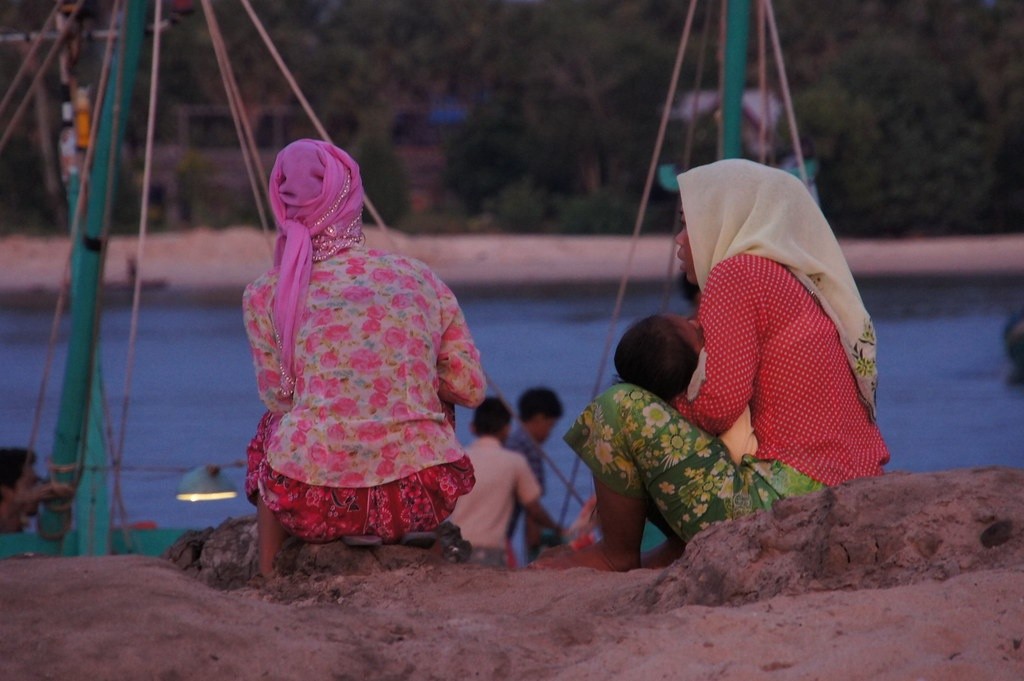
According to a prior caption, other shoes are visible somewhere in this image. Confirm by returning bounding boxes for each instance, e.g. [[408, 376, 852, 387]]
[[403, 530, 437, 548], [343, 534, 383, 547]]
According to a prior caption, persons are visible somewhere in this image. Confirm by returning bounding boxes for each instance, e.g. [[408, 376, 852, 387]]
[[528, 157, 889, 573], [1003, 310, 1024, 384], [507, 386, 563, 496], [0, 448, 39, 534], [445, 397, 569, 569], [613, 314, 757, 465], [244, 139, 489, 546]]
